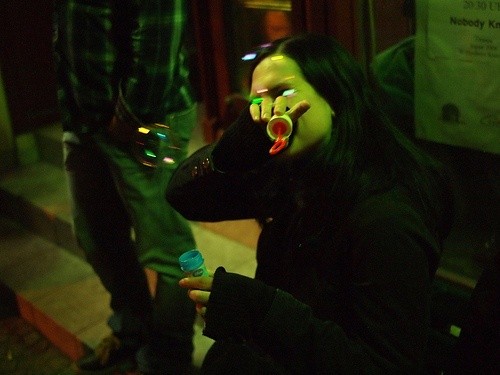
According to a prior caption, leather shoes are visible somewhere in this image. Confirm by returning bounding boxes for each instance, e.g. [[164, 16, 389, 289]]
[[77, 334, 137, 370]]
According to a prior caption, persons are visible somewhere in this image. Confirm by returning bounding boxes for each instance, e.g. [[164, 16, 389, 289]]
[[164, 29, 455, 375], [52, 0, 198, 375]]
